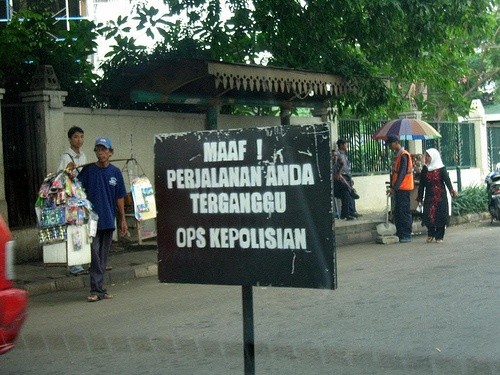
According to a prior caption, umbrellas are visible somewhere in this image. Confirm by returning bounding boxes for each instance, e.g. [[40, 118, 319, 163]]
[[372, 115, 442, 140]]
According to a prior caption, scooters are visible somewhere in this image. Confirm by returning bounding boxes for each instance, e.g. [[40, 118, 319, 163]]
[[484, 163, 500, 224]]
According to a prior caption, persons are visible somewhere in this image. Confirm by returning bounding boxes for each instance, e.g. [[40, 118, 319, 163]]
[[57, 125, 89, 276], [386, 135, 414, 243], [331, 138, 363, 221], [66, 137, 127, 302], [417, 148, 456, 243]]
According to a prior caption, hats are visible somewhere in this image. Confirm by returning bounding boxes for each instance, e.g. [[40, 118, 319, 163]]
[[96, 137, 112, 148]]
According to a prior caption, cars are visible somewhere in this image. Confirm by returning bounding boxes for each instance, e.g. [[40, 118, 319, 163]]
[[0, 213, 30, 357]]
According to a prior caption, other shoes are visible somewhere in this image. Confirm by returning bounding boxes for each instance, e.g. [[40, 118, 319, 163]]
[[88, 295, 97, 301], [427, 237, 433, 241], [437, 239, 440, 242], [104, 292, 113, 298]]
